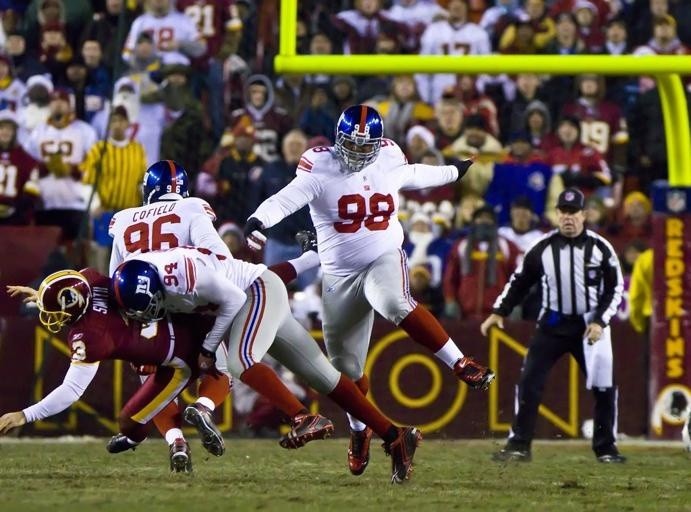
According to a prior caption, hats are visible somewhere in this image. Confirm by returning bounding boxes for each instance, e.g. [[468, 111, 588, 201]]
[[553, 187, 585, 210]]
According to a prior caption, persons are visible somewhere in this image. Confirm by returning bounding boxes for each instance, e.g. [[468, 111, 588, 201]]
[[479, 186, 628, 464], [241, 103, 495, 476], [110, 246, 422, 484], [2, 225, 321, 457], [0, 0, 691, 322], [107, 158, 234, 473]]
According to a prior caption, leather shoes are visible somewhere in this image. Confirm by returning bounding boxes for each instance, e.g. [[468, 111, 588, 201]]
[[491, 448, 531, 463], [597, 454, 626, 466]]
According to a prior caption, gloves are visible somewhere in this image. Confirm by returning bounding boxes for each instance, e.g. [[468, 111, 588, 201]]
[[243, 215, 267, 252], [452, 153, 477, 183]]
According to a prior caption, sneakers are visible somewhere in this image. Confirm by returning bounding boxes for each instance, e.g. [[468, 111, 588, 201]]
[[346, 420, 373, 475], [380, 425, 422, 486], [169, 437, 192, 474], [105, 433, 143, 453], [294, 225, 318, 252], [452, 354, 496, 392], [185, 400, 228, 459], [277, 413, 335, 450]]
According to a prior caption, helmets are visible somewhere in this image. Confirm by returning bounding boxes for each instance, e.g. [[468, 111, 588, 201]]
[[36, 269, 94, 334], [111, 259, 168, 323], [140, 159, 191, 202], [332, 104, 384, 173]]
